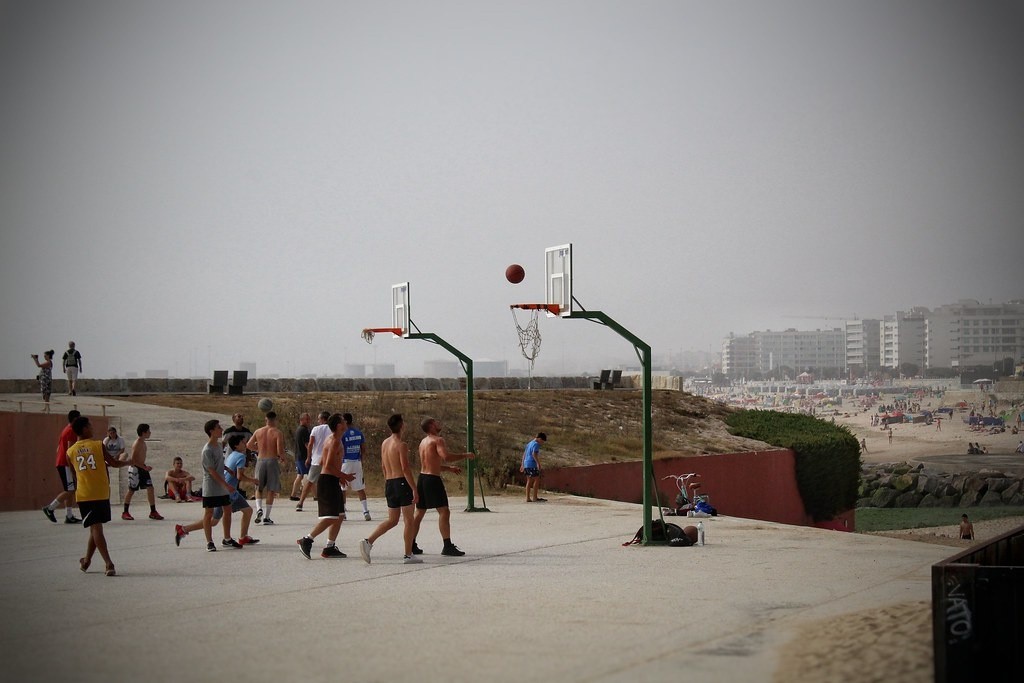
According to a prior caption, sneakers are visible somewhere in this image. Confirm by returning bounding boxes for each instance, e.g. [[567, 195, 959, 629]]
[[360, 538, 373, 564], [122, 511, 134, 520], [296, 535, 314, 559], [105, 565, 115, 576], [403, 554, 423, 564], [63, 515, 83, 524], [175, 508, 274, 552], [42, 506, 57, 523], [321, 543, 346, 558], [149, 511, 164, 520], [80, 558, 88, 572], [412, 547, 423, 554], [441, 543, 465, 556]]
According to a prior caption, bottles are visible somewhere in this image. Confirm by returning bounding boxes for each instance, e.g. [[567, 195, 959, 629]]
[[698, 521, 704, 545], [694, 496, 698, 504]]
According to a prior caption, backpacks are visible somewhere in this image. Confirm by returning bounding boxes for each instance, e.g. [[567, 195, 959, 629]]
[[65, 350, 77, 365]]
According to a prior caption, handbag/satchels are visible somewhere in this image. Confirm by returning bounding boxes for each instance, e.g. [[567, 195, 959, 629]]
[[664, 522, 692, 547], [622, 519, 667, 546]]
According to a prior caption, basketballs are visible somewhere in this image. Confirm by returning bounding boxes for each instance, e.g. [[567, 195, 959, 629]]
[[505, 264, 525, 284], [258, 397, 273, 413]]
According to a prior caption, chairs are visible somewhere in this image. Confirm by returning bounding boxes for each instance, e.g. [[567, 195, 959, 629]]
[[591, 370, 611, 390], [207, 370, 228, 394], [602, 370, 622, 390], [227, 370, 249, 394]]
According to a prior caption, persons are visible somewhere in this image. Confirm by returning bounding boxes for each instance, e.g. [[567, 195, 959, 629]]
[[102, 427, 128, 467], [859, 384, 1024, 456], [246, 412, 285, 525], [519, 432, 548, 503], [164, 456, 195, 503], [176, 434, 260, 547], [66, 416, 138, 576], [411, 417, 475, 557], [62, 341, 83, 396], [359, 414, 423, 564], [289, 412, 319, 501], [295, 410, 334, 511], [222, 412, 252, 460], [343, 412, 371, 521], [41, 410, 82, 525], [121, 424, 164, 521], [30, 349, 55, 413], [202, 420, 243, 552], [959, 514, 974, 540], [297, 412, 357, 560]]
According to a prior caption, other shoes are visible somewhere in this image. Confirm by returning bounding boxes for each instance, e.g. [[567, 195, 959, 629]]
[[176, 495, 181, 503], [364, 512, 371, 521], [69, 393, 71, 395], [289, 496, 300, 501], [314, 497, 318, 500], [296, 504, 302, 510], [72, 388, 75, 396], [185, 495, 193, 501]]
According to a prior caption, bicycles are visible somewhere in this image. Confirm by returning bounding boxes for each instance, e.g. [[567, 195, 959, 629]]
[[662, 472, 710, 505]]
